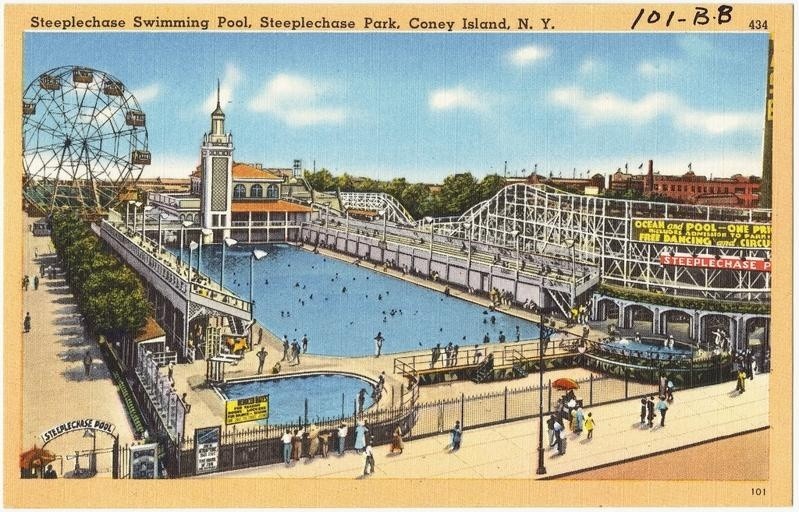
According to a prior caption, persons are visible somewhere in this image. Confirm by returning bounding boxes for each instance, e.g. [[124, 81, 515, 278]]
[[23, 218, 746, 479]]
